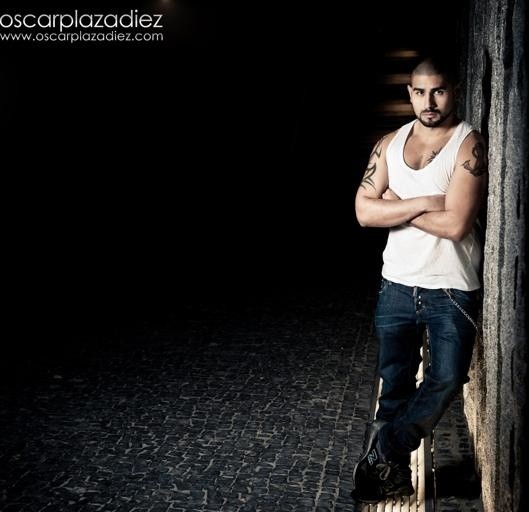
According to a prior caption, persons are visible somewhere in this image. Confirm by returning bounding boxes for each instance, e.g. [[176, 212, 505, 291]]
[[352, 58, 487, 504]]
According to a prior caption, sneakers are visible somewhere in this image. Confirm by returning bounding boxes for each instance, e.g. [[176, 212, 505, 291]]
[[353, 419, 392, 487], [350, 465, 415, 503]]
